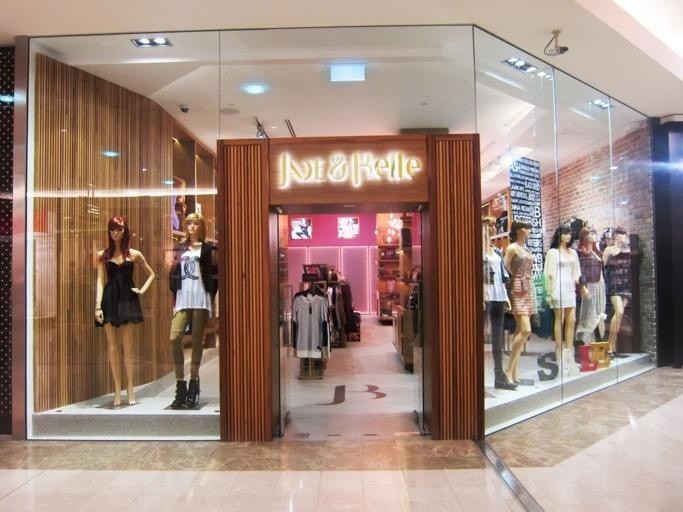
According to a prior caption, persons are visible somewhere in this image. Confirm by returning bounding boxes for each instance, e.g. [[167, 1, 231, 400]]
[[503, 220, 538, 388], [95, 217, 155, 406], [482, 217, 519, 390], [576, 226, 606, 345], [544, 225, 591, 377], [602, 228, 633, 357], [169, 214, 218, 408]]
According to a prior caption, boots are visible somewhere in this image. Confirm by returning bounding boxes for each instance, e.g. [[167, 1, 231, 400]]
[[494, 367, 518, 390], [180, 378, 200, 409], [171, 380, 188, 408]]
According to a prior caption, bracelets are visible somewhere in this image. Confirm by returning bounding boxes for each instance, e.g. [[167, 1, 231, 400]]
[[95, 308, 102, 313]]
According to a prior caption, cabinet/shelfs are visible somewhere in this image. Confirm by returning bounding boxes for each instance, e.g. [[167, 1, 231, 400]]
[[401, 265, 423, 346], [169, 118, 220, 345], [377, 244, 399, 320], [481, 186, 512, 241]]
